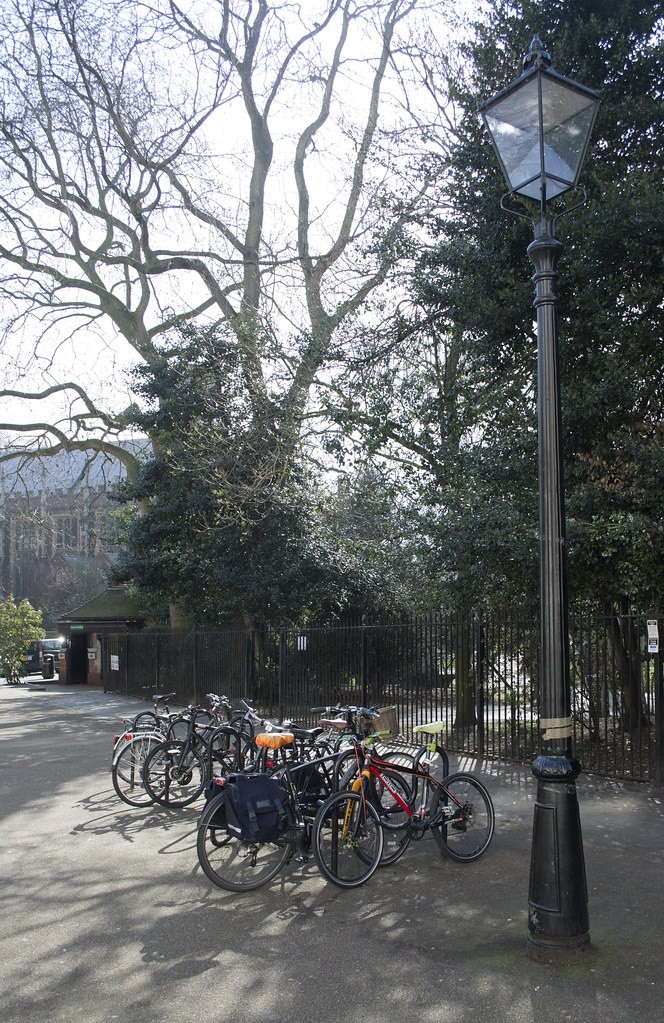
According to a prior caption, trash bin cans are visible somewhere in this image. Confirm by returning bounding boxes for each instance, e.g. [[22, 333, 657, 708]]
[[42, 653, 55, 679]]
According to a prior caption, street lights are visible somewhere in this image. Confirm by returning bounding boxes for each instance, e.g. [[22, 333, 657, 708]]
[[473, 33, 609, 964]]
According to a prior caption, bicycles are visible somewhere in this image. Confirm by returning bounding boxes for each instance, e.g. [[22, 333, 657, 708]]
[[107, 693, 499, 896]]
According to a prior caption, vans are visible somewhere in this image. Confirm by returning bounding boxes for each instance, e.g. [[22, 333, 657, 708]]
[[19, 639, 63, 672]]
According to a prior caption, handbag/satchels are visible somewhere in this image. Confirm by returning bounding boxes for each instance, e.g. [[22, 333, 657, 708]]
[[202, 779, 224, 825], [223, 776, 293, 843]]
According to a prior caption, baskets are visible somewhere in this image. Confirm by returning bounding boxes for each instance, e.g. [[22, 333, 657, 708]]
[[360, 706, 400, 740]]
